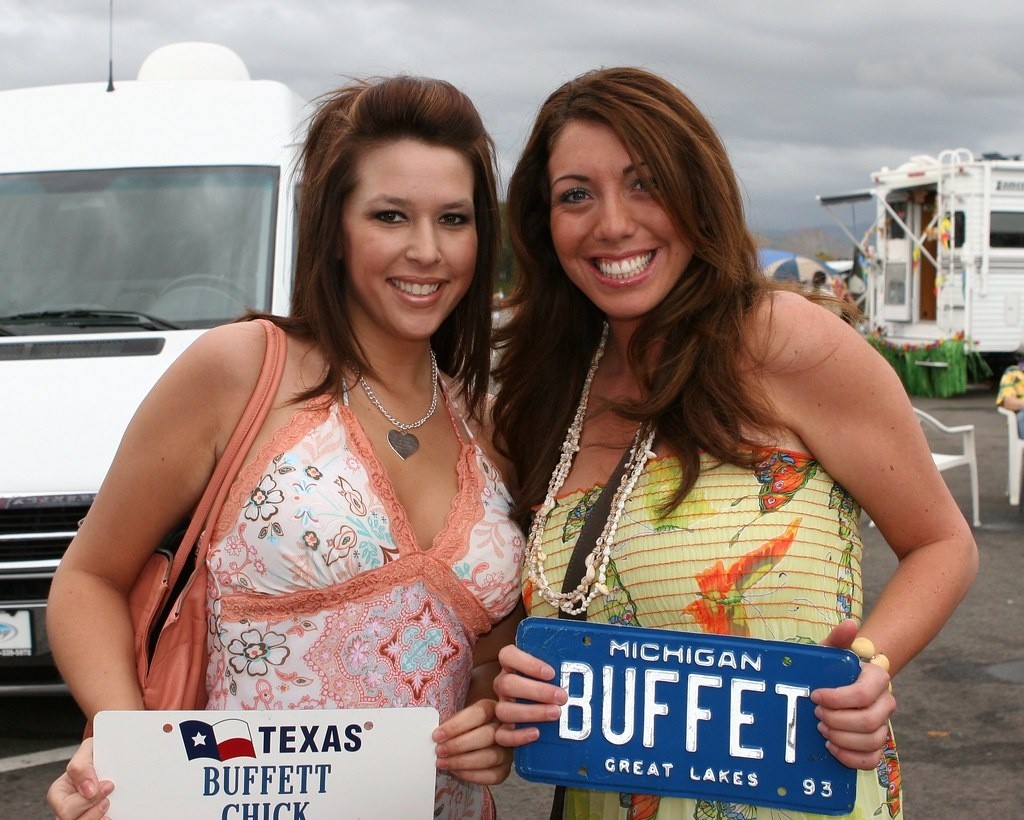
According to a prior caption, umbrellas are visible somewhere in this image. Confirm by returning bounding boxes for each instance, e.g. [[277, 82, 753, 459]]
[[750, 245, 843, 294]]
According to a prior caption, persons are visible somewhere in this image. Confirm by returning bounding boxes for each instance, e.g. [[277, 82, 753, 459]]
[[493, 65, 981, 820], [995, 342, 1024, 442], [44, 76, 530, 818], [810, 270, 841, 316]]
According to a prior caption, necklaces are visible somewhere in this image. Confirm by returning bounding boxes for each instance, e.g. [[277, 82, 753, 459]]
[[345, 344, 441, 460], [519, 317, 654, 617]]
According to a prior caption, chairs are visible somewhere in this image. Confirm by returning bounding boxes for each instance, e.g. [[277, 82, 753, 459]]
[[868, 408, 983, 527], [996, 405, 1024, 504]]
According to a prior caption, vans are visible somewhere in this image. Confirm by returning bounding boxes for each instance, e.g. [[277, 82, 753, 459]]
[[2, 40, 520, 695]]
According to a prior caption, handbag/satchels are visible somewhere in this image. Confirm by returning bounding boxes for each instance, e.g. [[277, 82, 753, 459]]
[[82, 318, 286, 746]]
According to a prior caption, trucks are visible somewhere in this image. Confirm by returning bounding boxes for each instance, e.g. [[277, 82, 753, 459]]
[[815, 149, 1024, 391]]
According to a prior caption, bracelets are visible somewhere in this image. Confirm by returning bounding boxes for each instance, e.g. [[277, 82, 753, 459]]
[[846, 635, 897, 703]]
